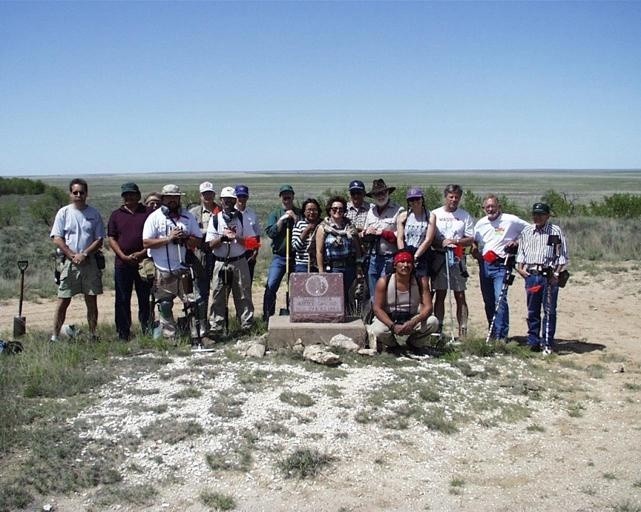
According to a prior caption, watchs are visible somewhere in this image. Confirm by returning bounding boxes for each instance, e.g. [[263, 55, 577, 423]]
[[83, 252, 88, 257]]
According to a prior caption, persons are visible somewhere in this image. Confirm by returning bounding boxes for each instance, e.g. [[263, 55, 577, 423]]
[[261, 178, 475, 356], [470, 196, 531, 341], [516, 203, 566, 355], [50, 178, 106, 343], [105, 182, 261, 346]]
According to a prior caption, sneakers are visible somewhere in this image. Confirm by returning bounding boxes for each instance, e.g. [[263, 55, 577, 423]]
[[539, 346, 552, 356], [192, 336, 214, 347]]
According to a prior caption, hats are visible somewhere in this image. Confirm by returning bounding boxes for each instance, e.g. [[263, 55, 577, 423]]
[[532, 202, 549, 214], [348, 180, 364, 191], [279, 185, 295, 196], [406, 187, 423, 199], [120, 182, 250, 204], [365, 178, 396, 198]]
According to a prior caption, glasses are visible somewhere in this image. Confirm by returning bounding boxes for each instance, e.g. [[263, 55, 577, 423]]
[[409, 197, 420, 202], [73, 191, 85, 195], [373, 190, 386, 196], [332, 207, 344, 212], [350, 189, 361, 195]]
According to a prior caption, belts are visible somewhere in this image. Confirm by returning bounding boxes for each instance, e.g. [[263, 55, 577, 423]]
[[528, 264, 548, 273], [218, 255, 244, 261]]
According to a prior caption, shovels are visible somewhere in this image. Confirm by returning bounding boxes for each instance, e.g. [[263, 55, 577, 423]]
[[13, 260, 29, 339], [279, 219, 290, 316], [177, 303, 190, 332]]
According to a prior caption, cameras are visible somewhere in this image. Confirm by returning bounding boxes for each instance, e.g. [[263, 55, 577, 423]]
[[392, 312, 410, 324], [173, 231, 189, 243], [406, 245, 418, 254]]
